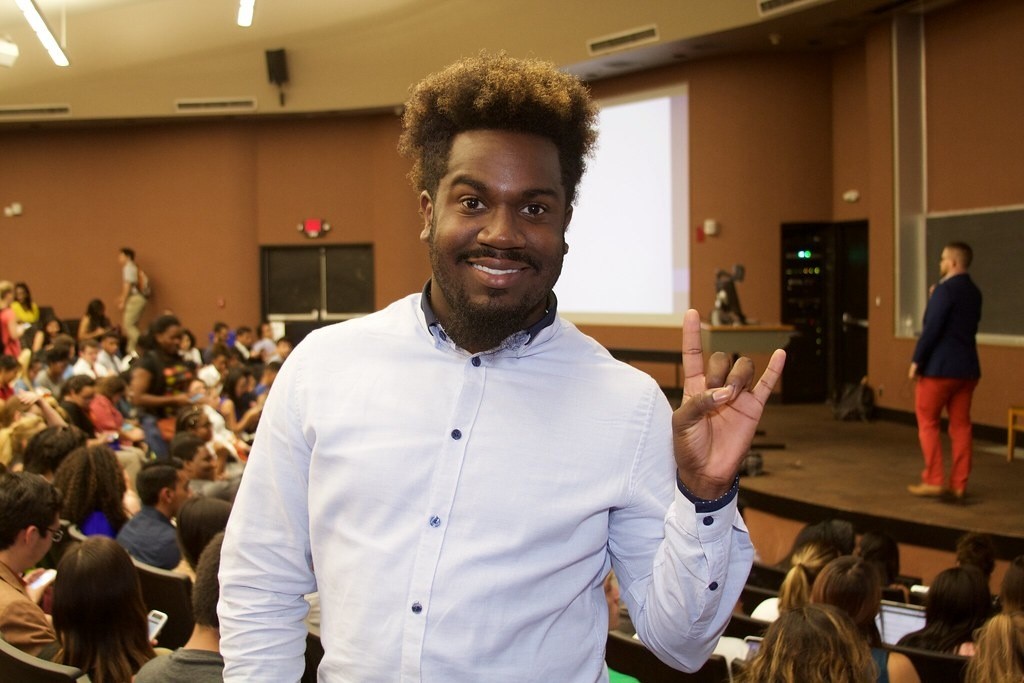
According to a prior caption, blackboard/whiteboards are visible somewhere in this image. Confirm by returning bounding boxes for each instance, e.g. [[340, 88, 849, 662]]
[[916, 204, 1023, 342]]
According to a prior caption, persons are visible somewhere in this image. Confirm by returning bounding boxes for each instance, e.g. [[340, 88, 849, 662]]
[[907, 241, 982, 498], [732, 520, 1024, 683], [1, 280, 292, 682], [604, 573, 641, 683], [44, 535, 177, 683], [117, 248, 148, 350], [117, 459, 193, 570], [217, 50, 786, 683]]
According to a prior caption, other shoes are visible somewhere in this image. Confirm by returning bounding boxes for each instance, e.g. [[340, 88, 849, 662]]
[[946, 485, 966, 501], [906, 481, 946, 497]]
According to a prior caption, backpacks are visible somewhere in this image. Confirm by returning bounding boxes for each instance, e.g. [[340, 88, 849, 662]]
[[132, 266, 152, 298]]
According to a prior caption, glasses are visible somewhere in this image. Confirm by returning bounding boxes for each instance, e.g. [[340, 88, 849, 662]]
[[40, 527, 65, 541]]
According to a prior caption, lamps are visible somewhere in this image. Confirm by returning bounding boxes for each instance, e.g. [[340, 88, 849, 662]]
[[14, 0, 70, 66], [295, 219, 330, 238]]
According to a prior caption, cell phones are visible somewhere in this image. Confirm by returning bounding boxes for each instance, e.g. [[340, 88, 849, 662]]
[[27, 568, 58, 590], [146, 609, 168, 641]]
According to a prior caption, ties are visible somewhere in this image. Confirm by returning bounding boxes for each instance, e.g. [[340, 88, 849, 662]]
[[90, 364, 98, 378], [110, 354, 122, 374]]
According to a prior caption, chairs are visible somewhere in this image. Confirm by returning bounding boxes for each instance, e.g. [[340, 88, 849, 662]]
[[1, 516, 973, 683], [1008, 407, 1024, 461]]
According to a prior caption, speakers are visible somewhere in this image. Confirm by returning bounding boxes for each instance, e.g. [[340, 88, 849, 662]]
[[267, 48, 287, 84]]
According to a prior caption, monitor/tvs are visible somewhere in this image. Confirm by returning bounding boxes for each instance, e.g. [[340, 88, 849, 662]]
[[716, 271, 745, 323]]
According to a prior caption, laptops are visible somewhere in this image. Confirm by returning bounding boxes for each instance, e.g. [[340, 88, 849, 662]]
[[874, 599, 928, 646]]
